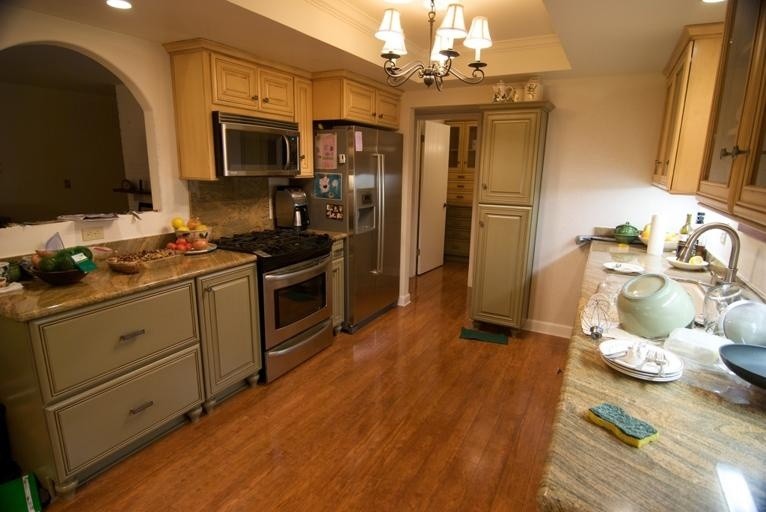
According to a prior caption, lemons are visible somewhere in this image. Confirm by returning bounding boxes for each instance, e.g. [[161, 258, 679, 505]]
[[172, 217, 207, 231]]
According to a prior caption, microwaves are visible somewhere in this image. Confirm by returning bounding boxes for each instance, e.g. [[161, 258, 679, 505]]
[[211, 110, 300, 178]]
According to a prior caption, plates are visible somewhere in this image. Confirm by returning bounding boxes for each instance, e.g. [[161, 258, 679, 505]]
[[665, 257, 708, 271], [638, 235, 677, 252], [183, 243, 217, 255], [598, 340, 684, 382], [603, 263, 644, 275]]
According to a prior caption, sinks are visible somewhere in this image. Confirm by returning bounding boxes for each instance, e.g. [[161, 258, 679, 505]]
[[664, 277, 707, 316]]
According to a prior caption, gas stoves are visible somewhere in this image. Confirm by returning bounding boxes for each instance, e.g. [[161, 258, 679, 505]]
[[207, 228, 334, 263]]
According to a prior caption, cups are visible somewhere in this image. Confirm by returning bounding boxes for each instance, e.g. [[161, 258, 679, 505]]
[[646, 215, 667, 255]]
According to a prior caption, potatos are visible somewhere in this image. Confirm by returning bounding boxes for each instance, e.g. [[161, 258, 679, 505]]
[[192, 240, 208, 249]]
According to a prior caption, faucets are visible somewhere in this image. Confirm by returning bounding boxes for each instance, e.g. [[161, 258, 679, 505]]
[[679, 222, 741, 281]]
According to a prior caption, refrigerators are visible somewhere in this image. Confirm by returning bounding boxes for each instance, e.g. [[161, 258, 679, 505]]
[[307, 124, 402, 334]]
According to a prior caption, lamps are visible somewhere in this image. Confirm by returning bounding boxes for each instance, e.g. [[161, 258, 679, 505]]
[[375, 1, 495, 94]]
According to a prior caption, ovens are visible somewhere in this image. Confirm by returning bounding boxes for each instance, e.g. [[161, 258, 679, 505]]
[[256, 256, 332, 384]]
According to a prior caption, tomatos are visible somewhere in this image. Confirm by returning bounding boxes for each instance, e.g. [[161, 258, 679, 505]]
[[167, 238, 192, 251]]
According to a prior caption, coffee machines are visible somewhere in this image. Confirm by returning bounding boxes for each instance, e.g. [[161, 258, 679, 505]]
[[274, 185, 309, 231]]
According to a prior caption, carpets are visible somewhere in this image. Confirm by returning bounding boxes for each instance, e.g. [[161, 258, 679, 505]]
[[459, 327, 509, 345]]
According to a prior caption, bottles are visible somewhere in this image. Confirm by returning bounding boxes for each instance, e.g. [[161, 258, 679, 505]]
[[692, 210, 706, 260], [676, 213, 693, 258]]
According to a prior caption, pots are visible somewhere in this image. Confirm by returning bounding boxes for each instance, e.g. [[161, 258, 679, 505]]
[[719, 344, 766, 390]]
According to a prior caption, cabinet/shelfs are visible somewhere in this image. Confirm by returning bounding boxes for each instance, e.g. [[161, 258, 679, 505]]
[[445, 206, 473, 257], [0, 279, 204, 501], [652, 21, 726, 198], [195, 261, 264, 417], [295, 65, 315, 180], [312, 69, 403, 131], [467, 203, 538, 333], [696, 1, 766, 233], [161, 36, 295, 122], [474, 101, 556, 207], [443, 119, 480, 208]]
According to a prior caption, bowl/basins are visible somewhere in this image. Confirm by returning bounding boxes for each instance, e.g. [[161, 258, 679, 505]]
[[30, 265, 88, 285], [617, 273, 695, 338], [703, 284, 752, 337], [613, 234, 637, 245], [0, 261, 29, 288], [174, 226, 212, 245]]
